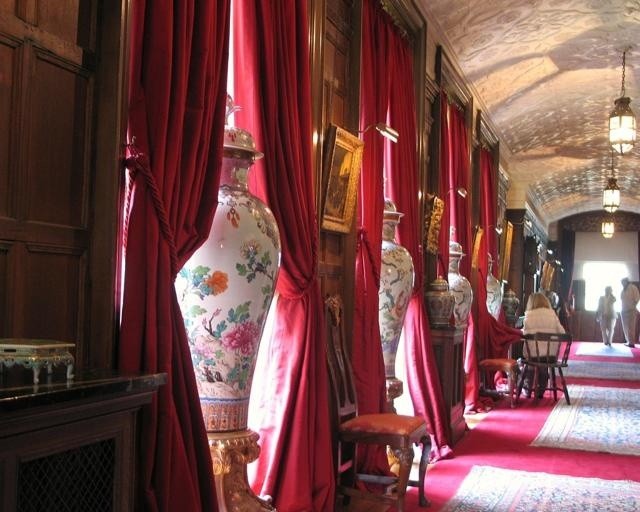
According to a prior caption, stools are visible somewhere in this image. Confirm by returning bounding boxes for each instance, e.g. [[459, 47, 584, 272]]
[[477, 358, 518, 409]]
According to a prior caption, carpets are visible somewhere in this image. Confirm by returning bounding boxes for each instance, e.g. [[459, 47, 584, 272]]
[[549, 359, 640, 381], [528, 383, 640, 456], [576, 340, 634, 358], [438, 464, 640, 511]]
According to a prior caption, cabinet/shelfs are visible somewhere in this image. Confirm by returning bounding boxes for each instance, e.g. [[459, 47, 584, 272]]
[[431, 327, 467, 446]]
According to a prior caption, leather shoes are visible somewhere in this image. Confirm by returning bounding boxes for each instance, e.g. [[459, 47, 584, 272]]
[[625, 343, 634, 347]]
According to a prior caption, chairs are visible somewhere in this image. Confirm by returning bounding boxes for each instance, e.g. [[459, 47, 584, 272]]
[[319, 292, 431, 511], [519, 332, 573, 408]]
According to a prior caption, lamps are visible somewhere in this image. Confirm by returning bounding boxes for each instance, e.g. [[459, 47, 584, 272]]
[[601, 215, 616, 240], [602, 147, 621, 215], [609, 51, 636, 157], [426, 187, 468, 198], [473, 224, 502, 236], [524, 217, 568, 273], [343, 120, 401, 144]]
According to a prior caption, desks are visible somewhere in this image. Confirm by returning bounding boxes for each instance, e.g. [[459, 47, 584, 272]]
[[0, 370, 169, 512]]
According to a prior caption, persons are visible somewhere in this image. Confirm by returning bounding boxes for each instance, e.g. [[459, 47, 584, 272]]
[[594, 286, 618, 348], [620, 277, 640, 349], [518, 291, 565, 397]]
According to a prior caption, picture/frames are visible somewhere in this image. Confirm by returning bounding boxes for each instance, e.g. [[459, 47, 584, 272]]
[[470, 224, 484, 271], [422, 192, 445, 257], [500, 220, 513, 286], [540, 261, 555, 291], [318, 122, 366, 235]]
